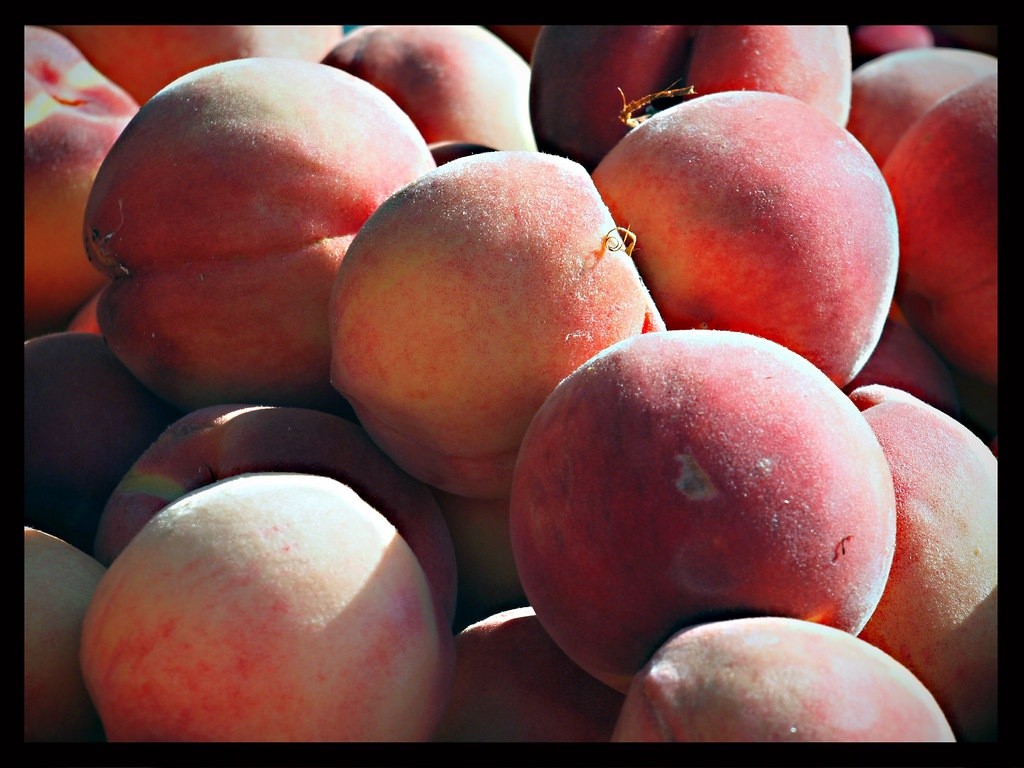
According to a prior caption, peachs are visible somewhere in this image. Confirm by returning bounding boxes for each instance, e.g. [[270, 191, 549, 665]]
[[25, 25, 998, 743]]
